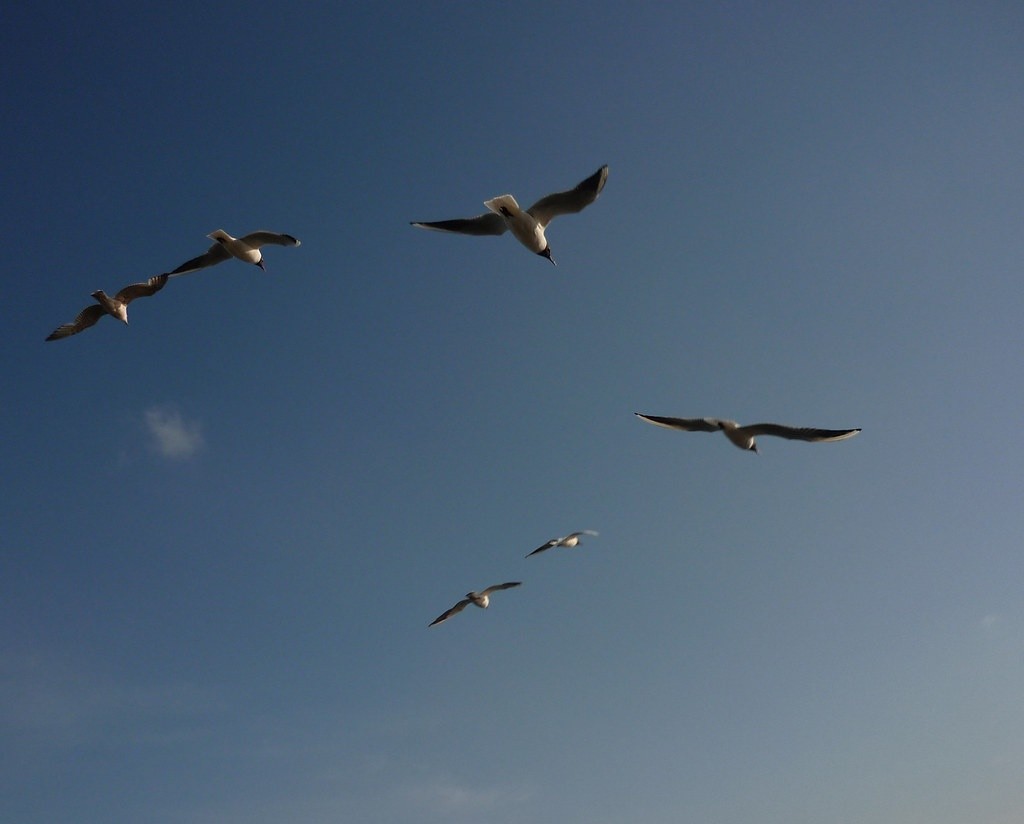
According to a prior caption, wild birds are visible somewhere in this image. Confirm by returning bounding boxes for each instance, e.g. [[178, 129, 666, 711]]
[[167, 228, 303, 273], [408, 163, 608, 267], [524, 530, 600, 559], [634, 412, 863, 456], [44, 273, 169, 342], [428, 581, 523, 627]]
